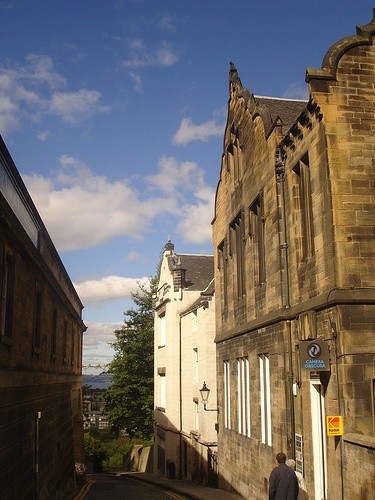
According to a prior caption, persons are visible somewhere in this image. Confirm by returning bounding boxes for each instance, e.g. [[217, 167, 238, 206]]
[[268, 452, 300, 500], [285, 458, 310, 500]]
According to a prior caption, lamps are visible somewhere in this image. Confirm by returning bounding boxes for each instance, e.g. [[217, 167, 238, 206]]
[[199, 381, 211, 404]]
